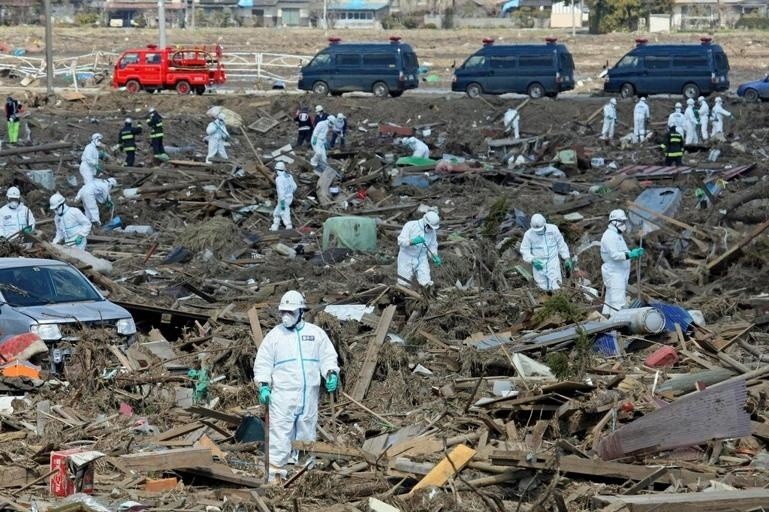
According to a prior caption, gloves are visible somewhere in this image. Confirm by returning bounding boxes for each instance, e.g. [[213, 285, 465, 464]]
[[281, 199, 286, 209], [432, 255, 442, 265], [326, 374, 339, 393], [224, 133, 228, 138], [215, 124, 220, 131], [105, 202, 112, 209], [23, 226, 32, 234], [627, 247, 644, 259], [95, 163, 103, 170], [412, 235, 425, 245], [532, 259, 542, 270], [260, 387, 272, 404], [98, 150, 107, 158], [75, 235, 84, 244], [312, 138, 318, 145], [564, 258, 571, 269]]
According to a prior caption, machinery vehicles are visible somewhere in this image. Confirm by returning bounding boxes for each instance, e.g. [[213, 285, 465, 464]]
[[111, 40, 226, 96]]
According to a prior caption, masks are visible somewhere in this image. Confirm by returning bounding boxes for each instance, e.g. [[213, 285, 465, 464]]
[[424, 225, 435, 233], [7, 200, 20, 208], [328, 122, 334, 127], [95, 141, 102, 146], [281, 312, 302, 328], [613, 222, 627, 233], [534, 228, 545, 235], [53, 206, 64, 217], [218, 120, 226, 125]]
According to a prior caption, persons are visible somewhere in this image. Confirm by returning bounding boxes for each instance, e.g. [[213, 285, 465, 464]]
[[601, 97, 618, 140], [697, 95, 711, 140], [631, 96, 651, 142], [658, 122, 686, 166], [72, 176, 122, 226], [117, 117, 142, 169], [330, 112, 349, 151], [309, 114, 339, 167], [47, 193, 94, 250], [667, 101, 685, 140], [503, 106, 521, 140], [518, 213, 572, 296], [708, 96, 734, 138], [253, 288, 342, 485], [203, 112, 231, 162], [683, 97, 701, 146], [312, 104, 331, 122], [3, 95, 23, 143], [396, 210, 442, 291], [0, 186, 36, 247], [143, 106, 172, 162], [78, 133, 103, 184], [400, 135, 431, 159], [294, 106, 313, 150], [268, 160, 298, 234], [599, 208, 646, 318]]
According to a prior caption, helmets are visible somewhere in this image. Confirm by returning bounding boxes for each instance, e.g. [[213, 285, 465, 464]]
[[274, 161, 286, 171], [5, 186, 21, 200], [609, 209, 630, 221], [609, 98, 617, 107], [675, 96, 723, 108], [91, 133, 105, 139], [107, 177, 119, 190], [299, 104, 345, 124], [530, 213, 547, 232], [50, 194, 66, 209], [401, 138, 408, 145], [149, 107, 155, 112], [7, 97, 14, 103], [424, 211, 441, 230], [218, 112, 227, 122], [638, 96, 646, 101], [124, 117, 133, 124], [668, 122, 676, 127], [278, 290, 308, 312]]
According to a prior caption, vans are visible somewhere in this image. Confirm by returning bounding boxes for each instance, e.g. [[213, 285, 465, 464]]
[[449, 37, 576, 100], [599, 40, 732, 100], [295, 41, 420, 100]]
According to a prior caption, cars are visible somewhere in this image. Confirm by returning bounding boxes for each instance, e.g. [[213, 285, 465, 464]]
[[0, 255, 138, 383], [735, 70, 769, 102]]
